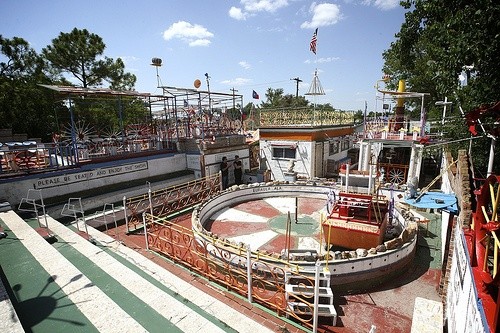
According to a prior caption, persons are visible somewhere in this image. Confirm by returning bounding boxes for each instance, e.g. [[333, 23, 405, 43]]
[[220, 156, 232, 190], [196, 117, 201, 124], [233, 155, 243, 185]]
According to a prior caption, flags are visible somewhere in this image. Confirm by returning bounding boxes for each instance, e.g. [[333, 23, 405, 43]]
[[309, 27, 318, 55], [252, 90, 259, 99]]
